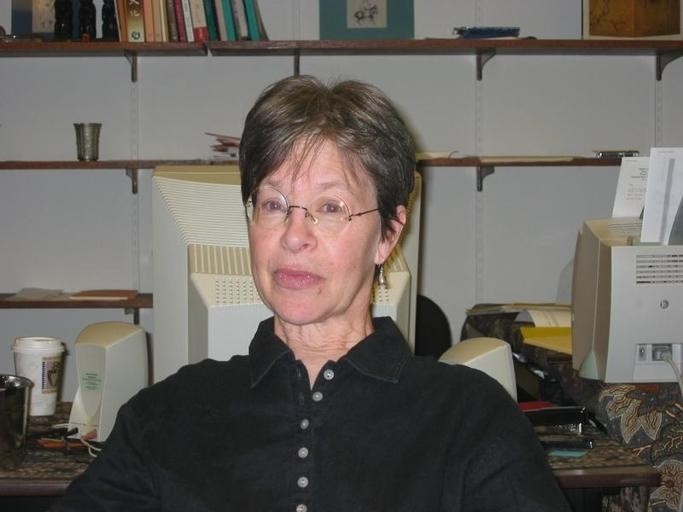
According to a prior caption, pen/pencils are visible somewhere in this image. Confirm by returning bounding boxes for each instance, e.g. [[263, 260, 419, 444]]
[[599, 152, 639, 157], [205, 133, 240, 152]]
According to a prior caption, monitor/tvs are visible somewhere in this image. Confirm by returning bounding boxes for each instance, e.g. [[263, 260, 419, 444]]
[[150, 164, 423, 386], [572, 216, 683, 384]]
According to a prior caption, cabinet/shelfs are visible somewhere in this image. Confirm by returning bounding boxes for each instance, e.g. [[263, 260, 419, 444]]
[[0, 37, 683, 325]]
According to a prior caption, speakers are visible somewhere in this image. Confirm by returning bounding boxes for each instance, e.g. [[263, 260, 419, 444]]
[[437, 336, 518, 406], [67, 320, 149, 442]]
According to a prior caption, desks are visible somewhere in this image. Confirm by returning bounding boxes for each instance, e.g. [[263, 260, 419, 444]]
[[1, 402, 662, 512]]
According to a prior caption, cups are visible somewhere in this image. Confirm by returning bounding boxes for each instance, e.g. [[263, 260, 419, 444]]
[[0, 374, 35, 470], [11, 336, 65, 416], [73, 122, 103, 162]]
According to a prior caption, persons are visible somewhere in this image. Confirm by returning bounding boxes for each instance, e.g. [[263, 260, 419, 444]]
[[56, 73, 578, 512]]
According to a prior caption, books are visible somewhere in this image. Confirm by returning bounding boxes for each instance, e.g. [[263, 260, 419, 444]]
[[112, 1, 269, 45], [9, 0, 56, 41]]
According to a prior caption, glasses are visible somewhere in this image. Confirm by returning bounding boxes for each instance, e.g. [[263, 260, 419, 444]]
[[246, 187, 382, 233]]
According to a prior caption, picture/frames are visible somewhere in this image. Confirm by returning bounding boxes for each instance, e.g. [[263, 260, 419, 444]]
[[581, 0, 683, 40]]
